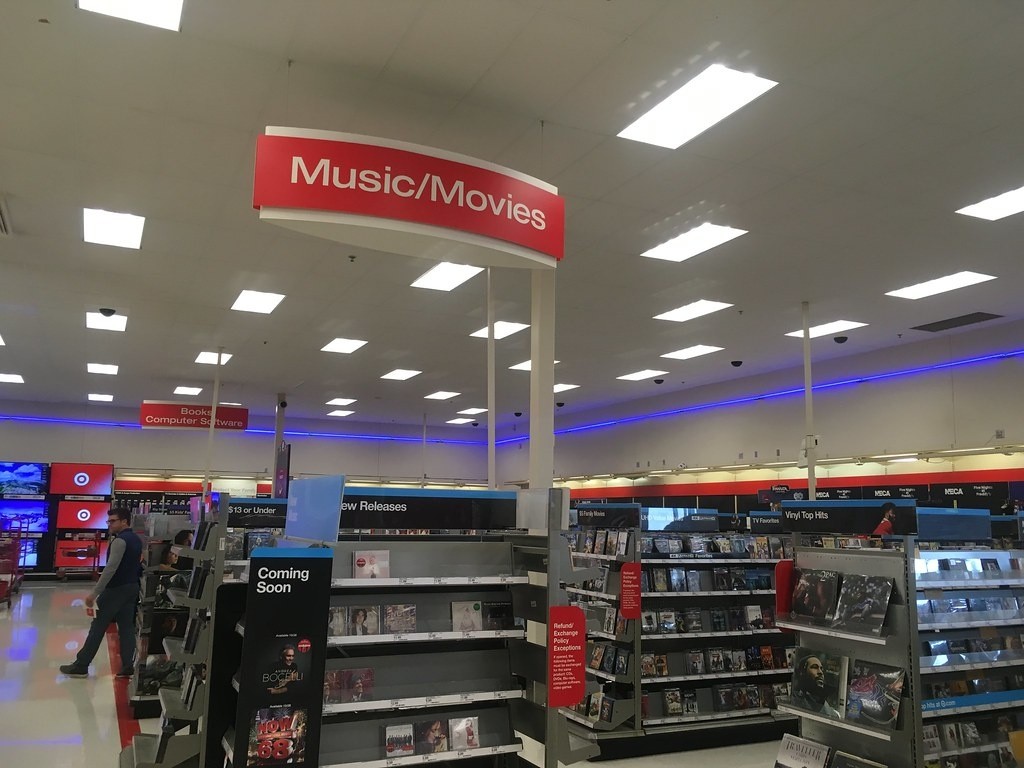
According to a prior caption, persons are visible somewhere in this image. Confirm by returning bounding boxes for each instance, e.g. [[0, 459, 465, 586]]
[[266, 642, 304, 699], [350, 675, 374, 702], [798, 653, 841, 719], [352, 609, 368, 635], [872, 502, 898, 536], [159, 544, 180, 572], [417, 720, 446, 755], [387, 732, 412, 749], [590, 645, 601, 668], [60, 507, 143, 679], [174, 530, 194, 549]]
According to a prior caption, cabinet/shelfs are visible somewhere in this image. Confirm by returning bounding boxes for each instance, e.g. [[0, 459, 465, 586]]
[[110, 486, 1024, 768]]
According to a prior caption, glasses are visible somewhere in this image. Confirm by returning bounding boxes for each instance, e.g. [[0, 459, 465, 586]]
[[105, 518, 121, 524], [284, 654, 294, 657]]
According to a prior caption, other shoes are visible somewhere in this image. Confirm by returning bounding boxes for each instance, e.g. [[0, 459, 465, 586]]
[[115, 669, 135, 678], [59, 660, 89, 678]]
